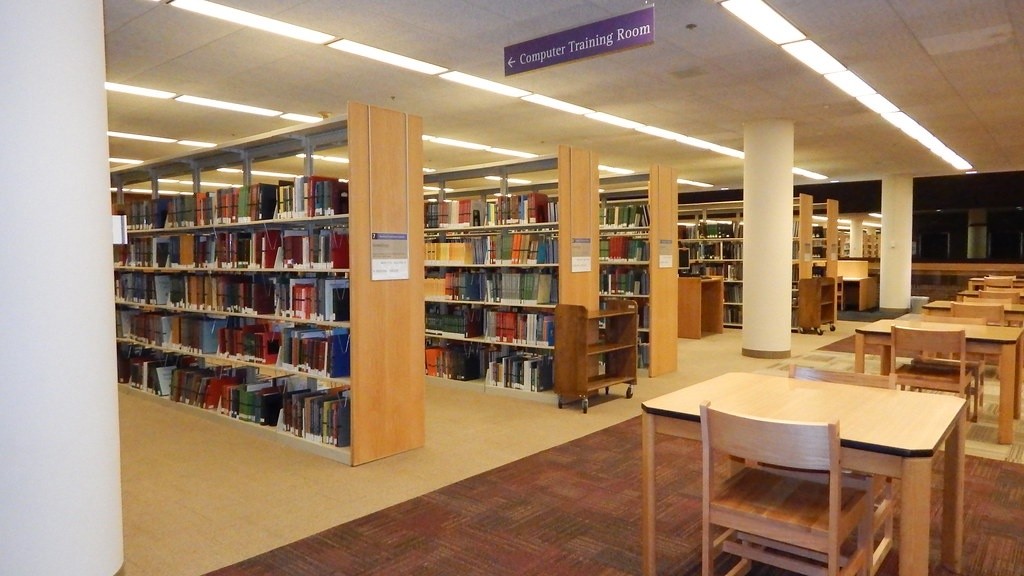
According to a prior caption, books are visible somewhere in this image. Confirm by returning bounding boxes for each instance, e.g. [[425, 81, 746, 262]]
[[111, 175, 350, 448], [677, 224, 743, 324], [810, 226, 827, 277], [792, 220, 800, 329], [599, 204, 650, 368], [423, 192, 561, 393]]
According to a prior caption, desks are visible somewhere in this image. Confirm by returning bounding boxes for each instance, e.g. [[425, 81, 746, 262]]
[[968, 278, 1024, 291], [855, 319, 1024, 445], [679, 276, 724, 338], [956, 288, 1024, 304], [641, 371, 966, 576], [924, 301, 1024, 328]]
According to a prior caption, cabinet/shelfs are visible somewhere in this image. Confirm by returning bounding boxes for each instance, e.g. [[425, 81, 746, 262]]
[[678, 199, 838, 277], [110, 103, 425, 466], [797, 277, 836, 335], [423, 145, 600, 405], [423, 160, 678, 377], [678, 193, 814, 331], [554, 300, 638, 414]]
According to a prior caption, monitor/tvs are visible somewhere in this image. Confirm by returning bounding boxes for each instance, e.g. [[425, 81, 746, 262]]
[[679, 248, 690, 269]]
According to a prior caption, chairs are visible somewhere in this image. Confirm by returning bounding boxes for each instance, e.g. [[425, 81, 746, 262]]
[[700, 273, 1017, 576]]
